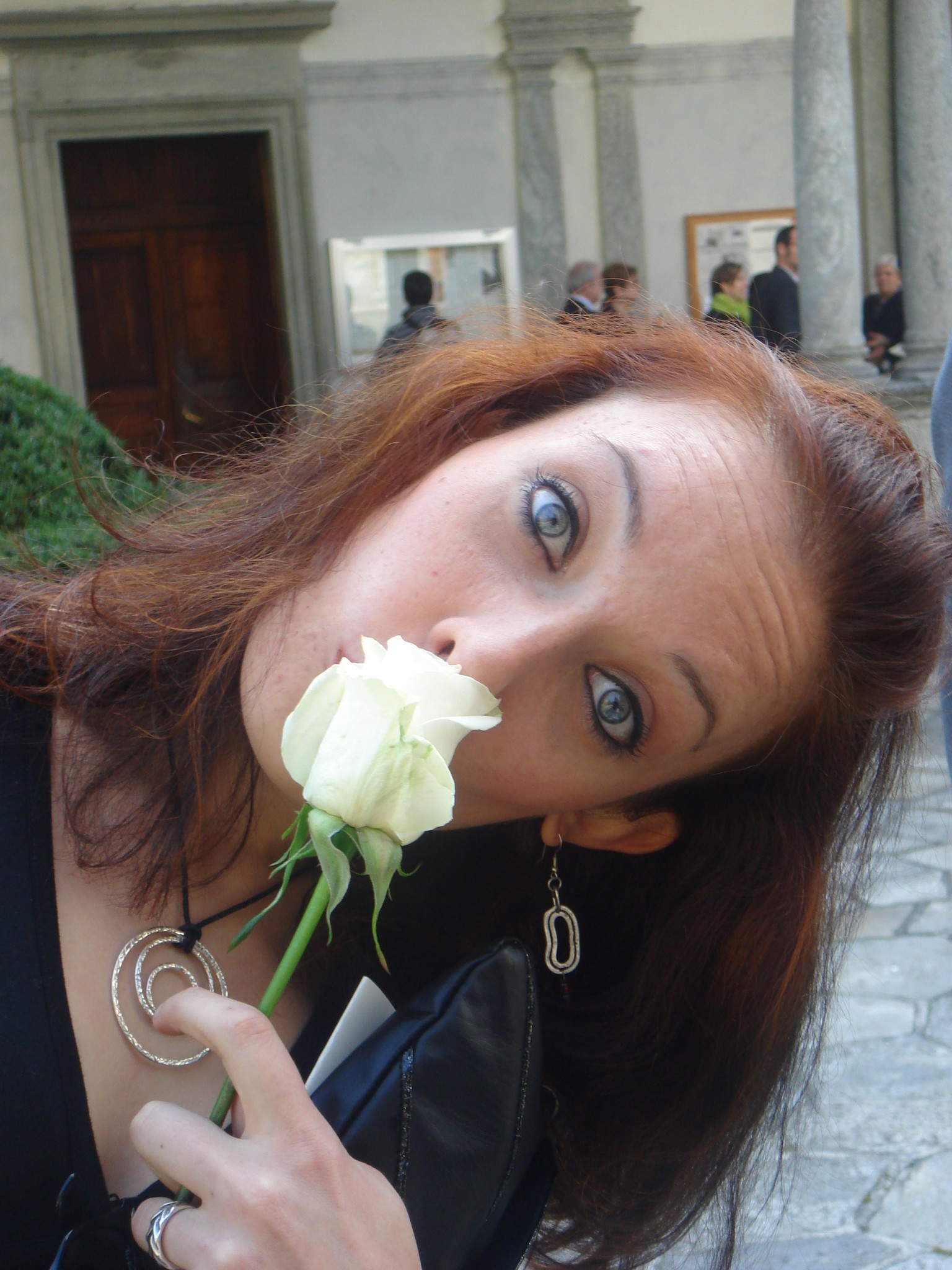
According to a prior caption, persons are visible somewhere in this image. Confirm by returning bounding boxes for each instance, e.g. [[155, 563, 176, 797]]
[[0, 226, 952, 1270]]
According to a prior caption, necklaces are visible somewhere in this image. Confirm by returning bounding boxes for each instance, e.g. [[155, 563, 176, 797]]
[[112, 723, 323, 1067]]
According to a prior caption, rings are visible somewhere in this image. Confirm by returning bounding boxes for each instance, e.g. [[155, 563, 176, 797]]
[[144, 1200, 197, 1270]]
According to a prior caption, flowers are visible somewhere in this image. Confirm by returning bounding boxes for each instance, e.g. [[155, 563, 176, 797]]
[[160, 629, 508, 1196]]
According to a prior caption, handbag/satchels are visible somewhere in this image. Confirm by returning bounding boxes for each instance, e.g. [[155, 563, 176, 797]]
[[310, 937, 562, 1270]]
